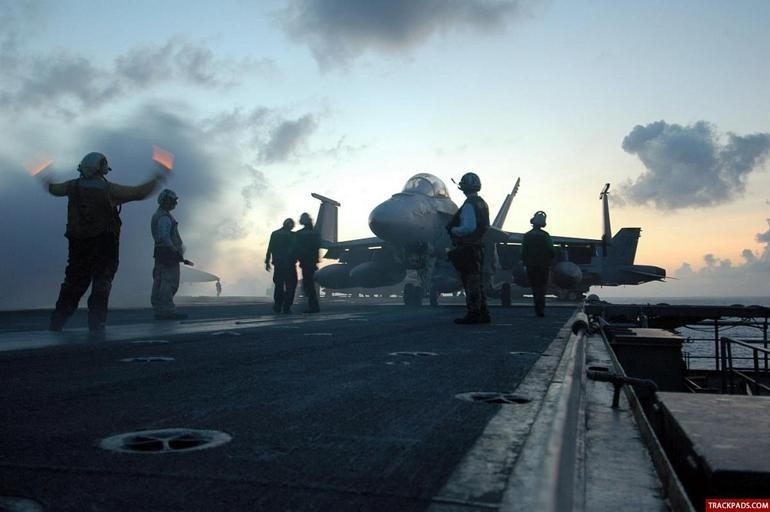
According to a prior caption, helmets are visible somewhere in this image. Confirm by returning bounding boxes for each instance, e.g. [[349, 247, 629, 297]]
[[458, 173, 481, 192], [530, 211, 546, 227], [78, 152, 109, 173], [158, 190, 178, 204]]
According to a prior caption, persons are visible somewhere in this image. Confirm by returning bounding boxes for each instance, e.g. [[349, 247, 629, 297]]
[[216, 280, 221, 297], [445, 173, 490, 323], [151, 188, 185, 319], [48, 153, 162, 331], [265, 217, 299, 315], [297, 213, 320, 313], [521, 211, 555, 318]]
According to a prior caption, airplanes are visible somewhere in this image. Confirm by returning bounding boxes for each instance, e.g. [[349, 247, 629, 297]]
[[312, 173, 667, 308]]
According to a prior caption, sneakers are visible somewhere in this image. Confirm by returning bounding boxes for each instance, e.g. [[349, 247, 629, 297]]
[[454, 311, 491, 323]]
